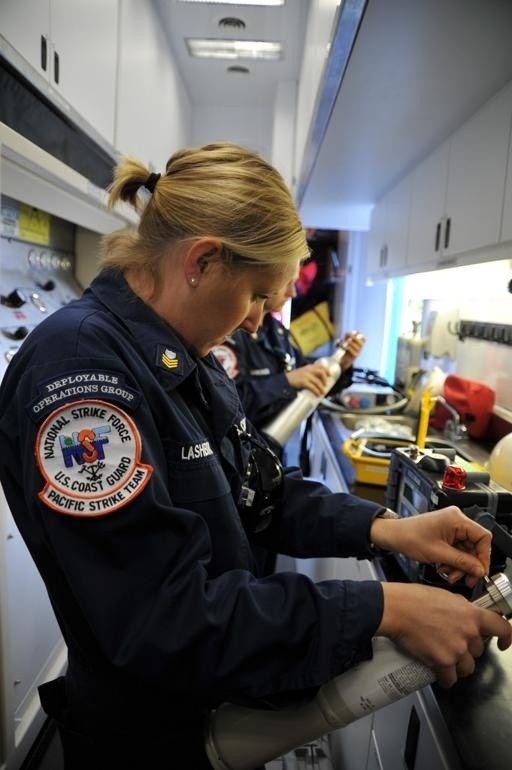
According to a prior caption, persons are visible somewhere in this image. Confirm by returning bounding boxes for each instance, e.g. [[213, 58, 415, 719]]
[[0, 142, 511, 770], [210, 260, 365, 430]]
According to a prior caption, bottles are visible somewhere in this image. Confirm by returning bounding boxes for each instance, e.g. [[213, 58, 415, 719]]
[[202, 573, 512, 768], [394, 321, 425, 388], [255, 332, 360, 454]]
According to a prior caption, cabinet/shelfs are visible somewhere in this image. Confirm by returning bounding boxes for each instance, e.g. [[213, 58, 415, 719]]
[[0, 0, 119, 146], [408, 83, 511, 268], [368, 174, 408, 273]]
